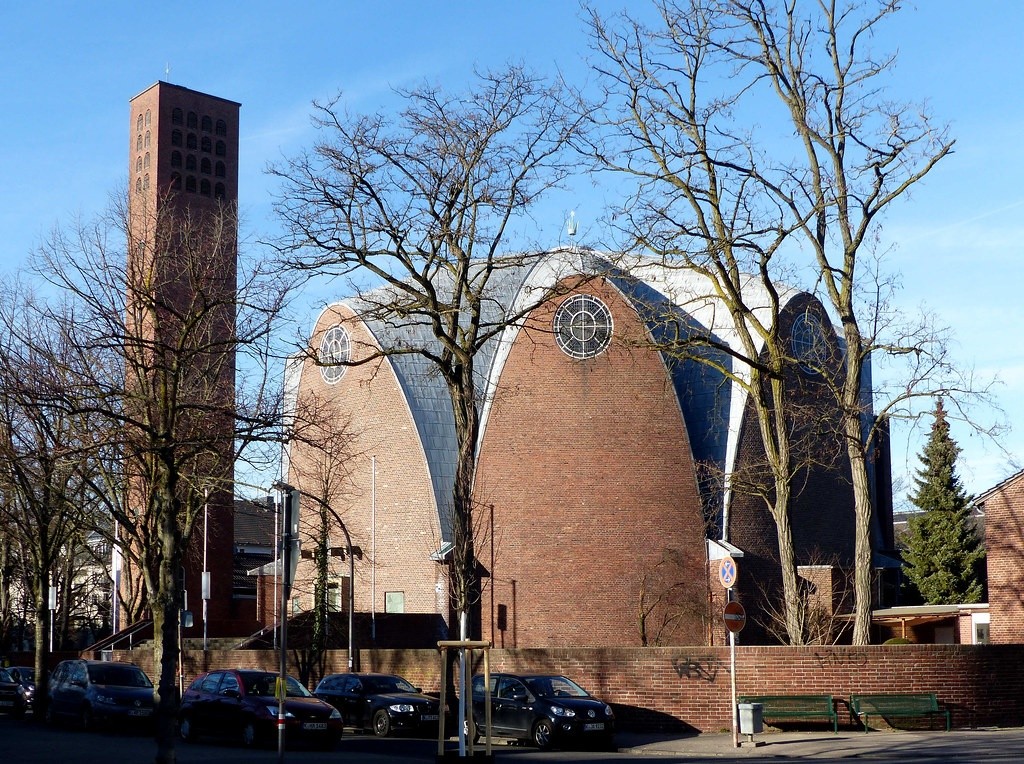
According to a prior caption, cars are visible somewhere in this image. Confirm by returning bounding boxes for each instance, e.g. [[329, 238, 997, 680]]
[[176, 668, 342, 749], [456, 672, 616, 755], [313, 673, 451, 738], [0, 666, 51, 724]]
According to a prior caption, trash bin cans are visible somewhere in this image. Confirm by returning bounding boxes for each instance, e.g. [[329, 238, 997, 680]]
[[738, 702, 763, 734]]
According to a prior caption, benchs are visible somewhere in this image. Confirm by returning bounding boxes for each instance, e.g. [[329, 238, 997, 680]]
[[739, 694, 837, 736], [849, 694, 950, 734]]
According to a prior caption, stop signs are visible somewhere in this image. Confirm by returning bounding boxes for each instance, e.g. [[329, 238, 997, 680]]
[[722, 601, 746, 633]]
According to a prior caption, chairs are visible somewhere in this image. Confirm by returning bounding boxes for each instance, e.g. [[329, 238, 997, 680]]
[[256, 681, 270, 696], [377, 680, 388, 688]]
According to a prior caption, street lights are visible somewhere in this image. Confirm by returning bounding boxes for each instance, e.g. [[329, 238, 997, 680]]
[[273, 481, 354, 676]]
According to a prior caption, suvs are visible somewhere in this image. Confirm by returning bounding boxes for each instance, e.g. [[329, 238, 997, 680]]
[[45, 658, 157, 733]]
